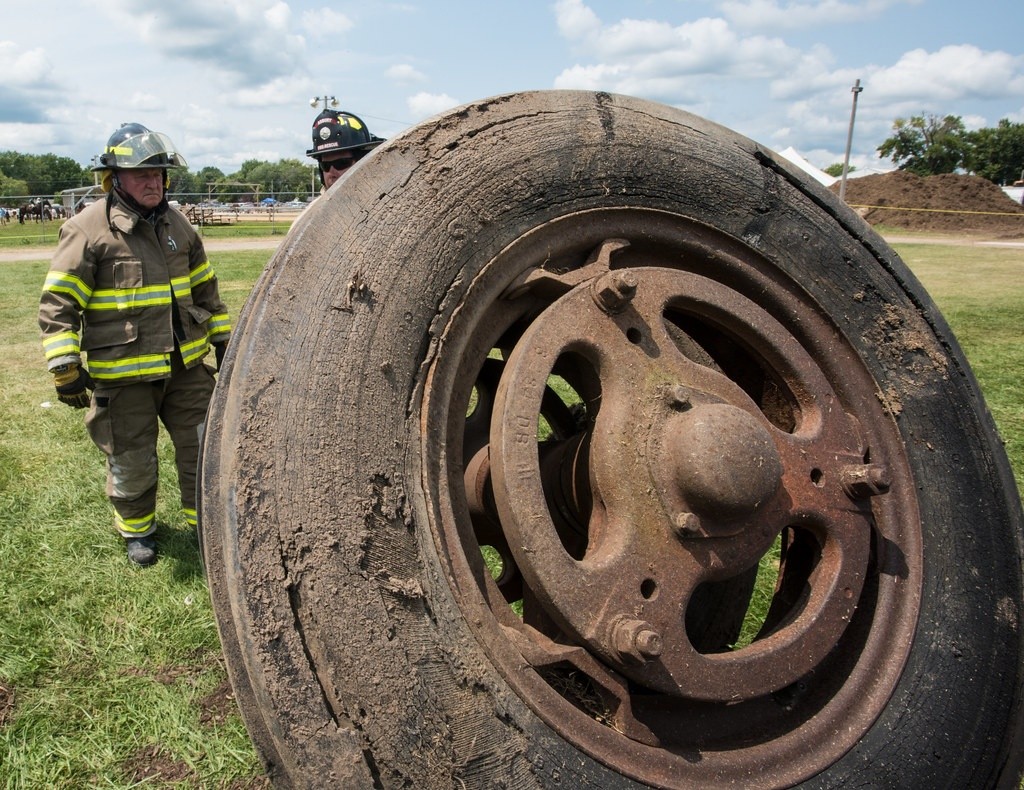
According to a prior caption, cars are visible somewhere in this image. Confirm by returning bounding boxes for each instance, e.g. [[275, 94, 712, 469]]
[[261, 198, 277, 204]]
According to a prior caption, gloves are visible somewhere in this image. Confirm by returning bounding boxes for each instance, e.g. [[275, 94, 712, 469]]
[[51, 364, 96, 409], [211, 340, 229, 372]]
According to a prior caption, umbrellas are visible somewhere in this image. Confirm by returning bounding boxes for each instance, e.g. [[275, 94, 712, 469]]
[[263, 198, 276, 203]]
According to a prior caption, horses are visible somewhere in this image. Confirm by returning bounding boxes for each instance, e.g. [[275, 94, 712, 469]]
[[19, 199, 53, 225]]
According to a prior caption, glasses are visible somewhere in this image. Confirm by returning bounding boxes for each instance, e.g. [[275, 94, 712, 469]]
[[320, 157, 361, 172]]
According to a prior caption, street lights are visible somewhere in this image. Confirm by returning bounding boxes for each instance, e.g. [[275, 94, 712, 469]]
[[310, 94, 339, 110]]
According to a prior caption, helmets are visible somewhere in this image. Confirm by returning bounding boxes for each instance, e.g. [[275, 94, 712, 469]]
[[306, 109, 387, 157], [90, 123, 179, 172]]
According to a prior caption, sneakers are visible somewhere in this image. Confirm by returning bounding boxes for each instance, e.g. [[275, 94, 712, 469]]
[[126, 533, 156, 567]]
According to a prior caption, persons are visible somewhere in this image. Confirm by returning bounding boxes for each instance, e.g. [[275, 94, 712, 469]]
[[1, 204, 13, 225], [38, 122, 232, 566], [307, 107, 386, 190]]
